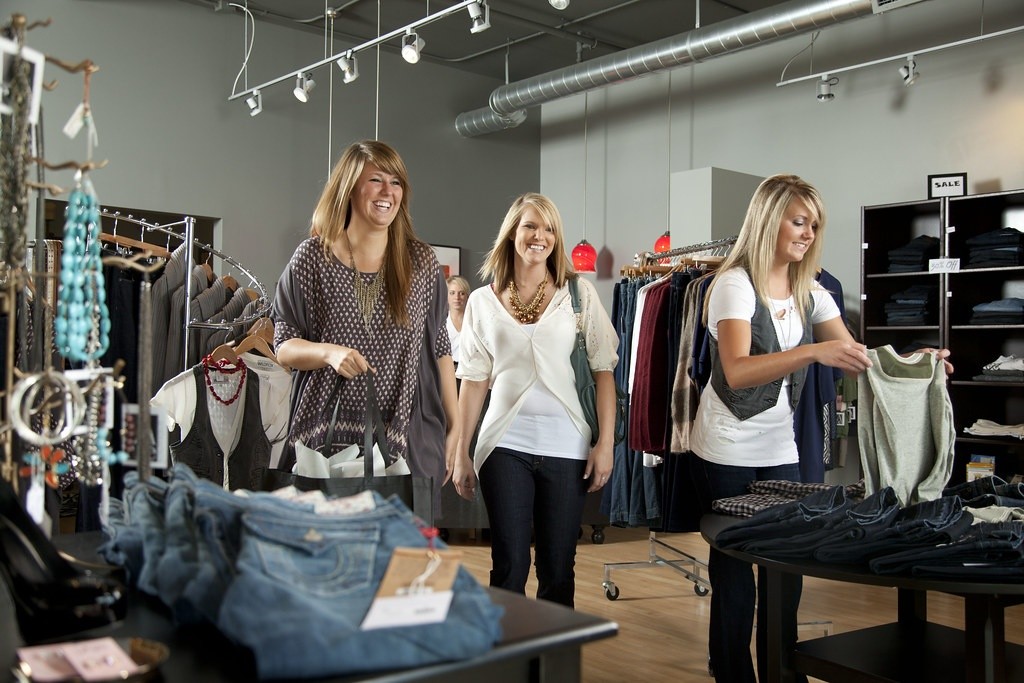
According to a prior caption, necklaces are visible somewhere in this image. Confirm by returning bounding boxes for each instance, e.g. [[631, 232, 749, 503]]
[[769, 290, 792, 351], [346, 233, 387, 337], [507, 268, 548, 323], [201, 353, 245, 405], [1, 70, 114, 487]]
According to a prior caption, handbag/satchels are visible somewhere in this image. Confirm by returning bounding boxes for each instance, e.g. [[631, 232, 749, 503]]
[[289, 366, 414, 514], [567, 275, 627, 448]]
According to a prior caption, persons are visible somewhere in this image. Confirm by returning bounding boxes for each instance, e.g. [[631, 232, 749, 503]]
[[689, 174, 953, 683], [273, 138, 460, 521], [452, 192, 620, 613], [443, 275, 471, 396]]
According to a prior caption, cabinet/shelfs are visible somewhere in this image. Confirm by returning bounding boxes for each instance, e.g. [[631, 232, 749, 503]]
[[858, 186, 1024, 484], [0, 13, 115, 579]]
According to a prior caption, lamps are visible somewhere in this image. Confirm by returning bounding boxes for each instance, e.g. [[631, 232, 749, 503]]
[[467, 0, 492, 33], [572, 92, 597, 272], [246, 90, 262, 116], [815, 75, 835, 103], [401, 27, 426, 64], [654, 71, 671, 264], [548, 0, 570, 11], [337, 55, 361, 84], [294, 72, 315, 104], [898, 55, 920, 88]]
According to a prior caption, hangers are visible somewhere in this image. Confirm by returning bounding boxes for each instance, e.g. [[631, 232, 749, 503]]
[[102, 212, 293, 390], [620, 236, 738, 277]]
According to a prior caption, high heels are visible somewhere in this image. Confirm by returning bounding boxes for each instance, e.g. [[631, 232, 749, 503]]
[[0, 472, 127, 645]]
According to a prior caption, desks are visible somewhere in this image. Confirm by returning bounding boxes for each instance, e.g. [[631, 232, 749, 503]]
[[700, 527, 1024, 683], [26, 529, 619, 683]]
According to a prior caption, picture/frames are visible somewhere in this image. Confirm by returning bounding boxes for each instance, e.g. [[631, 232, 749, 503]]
[[928, 172, 967, 200], [428, 244, 461, 277]]
